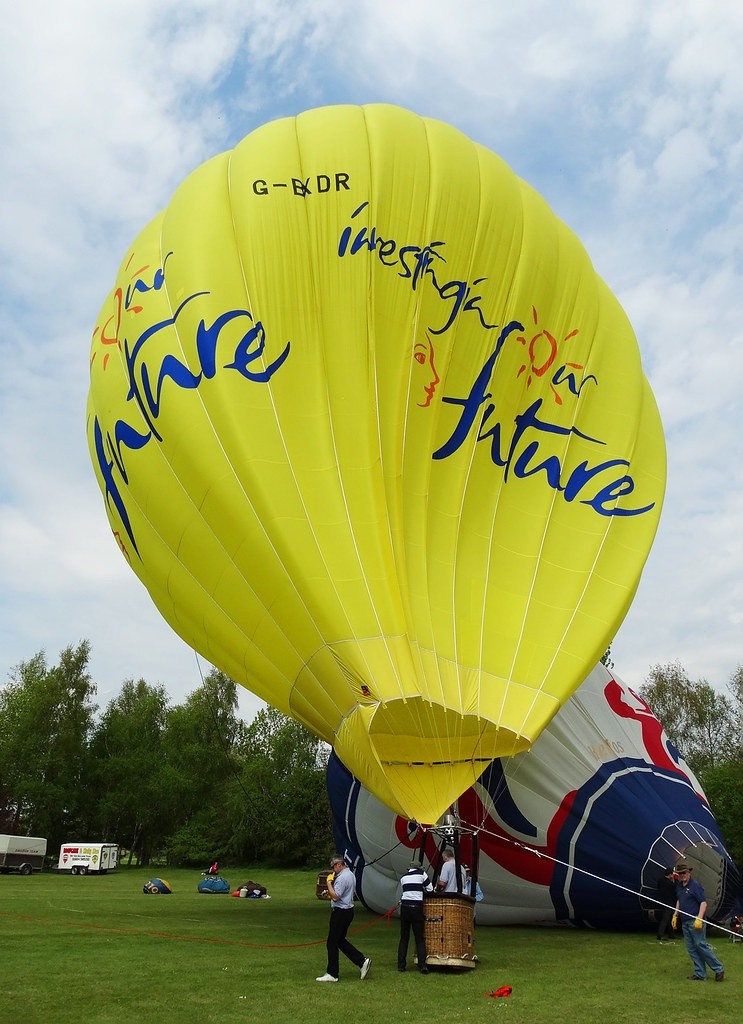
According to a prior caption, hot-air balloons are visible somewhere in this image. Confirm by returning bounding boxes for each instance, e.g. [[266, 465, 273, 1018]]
[[82, 103, 671, 974], [326, 659, 743, 932]]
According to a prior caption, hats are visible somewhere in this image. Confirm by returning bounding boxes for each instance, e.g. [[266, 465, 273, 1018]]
[[673, 864, 693, 873], [406, 861, 426, 870], [463, 864, 470, 871]]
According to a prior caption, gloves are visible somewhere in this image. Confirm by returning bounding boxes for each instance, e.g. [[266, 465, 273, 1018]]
[[693, 917, 702, 928], [672, 916, 677, 928], [326, 872, 335, 882]]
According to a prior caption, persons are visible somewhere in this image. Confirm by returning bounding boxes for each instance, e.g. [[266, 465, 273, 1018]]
[[671, 863, 725, 982], [433, 848, 484, 929], [397, 862, 434, 975], [656, 867, 677, 941], [315, 853, 372, 982], [209, 862, 219, 875]]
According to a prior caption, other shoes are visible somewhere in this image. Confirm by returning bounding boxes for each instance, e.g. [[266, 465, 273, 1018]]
[[657, 936, 669, 941], [360, 959, 371, 980], [316, 973, 338, 982], [669, 935, 677, 939], [398, 967, 406, 971], [687, 975, 703, 981], [422, 967, 428, 974], [716, 971, 724, 982]]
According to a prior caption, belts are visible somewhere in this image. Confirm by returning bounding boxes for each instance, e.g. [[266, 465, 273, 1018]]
[[402, 904, 422, 908], [331, 907, 353, 911]]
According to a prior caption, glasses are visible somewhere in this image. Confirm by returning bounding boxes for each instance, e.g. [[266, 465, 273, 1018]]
[[331, 863, 338, 869], [678, 871, 690, 875]]
